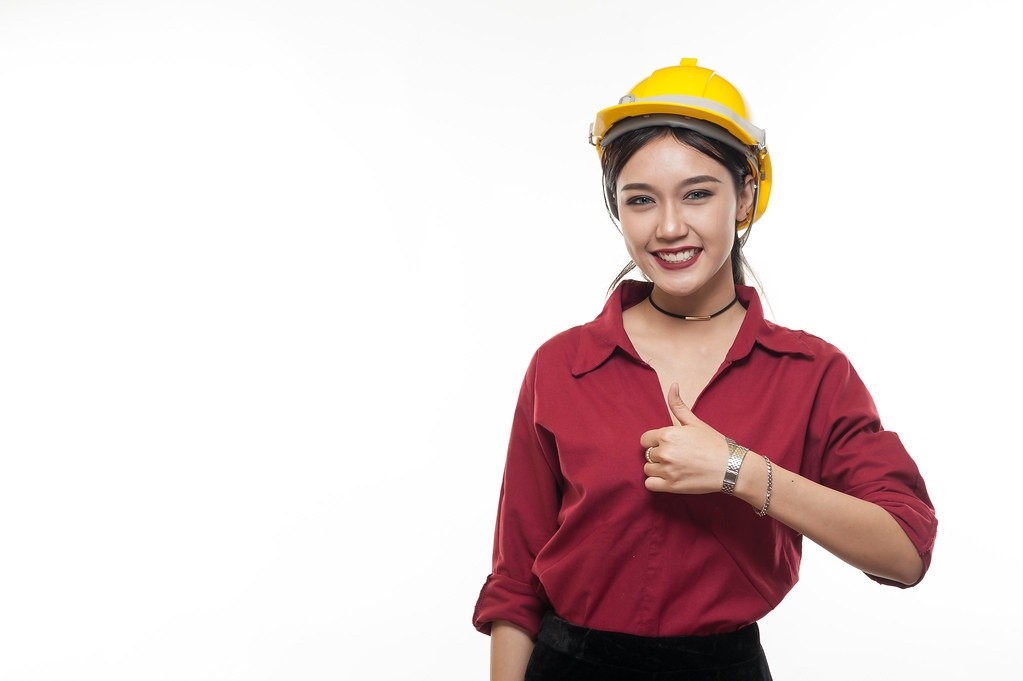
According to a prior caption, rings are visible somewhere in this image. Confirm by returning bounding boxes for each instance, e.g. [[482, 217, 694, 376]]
[[645, 447, 653, 464]]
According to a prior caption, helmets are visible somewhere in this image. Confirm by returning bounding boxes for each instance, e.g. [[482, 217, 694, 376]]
[[589, 57, 773, 231]]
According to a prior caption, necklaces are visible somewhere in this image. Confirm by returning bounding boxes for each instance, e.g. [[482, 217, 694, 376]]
[[646, 292, 739, 322]]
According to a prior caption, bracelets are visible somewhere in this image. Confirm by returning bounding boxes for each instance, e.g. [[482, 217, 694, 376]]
[[721, 437, 749, 495], [751, 455, 772, 517]]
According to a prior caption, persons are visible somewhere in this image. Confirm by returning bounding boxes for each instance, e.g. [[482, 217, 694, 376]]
[[472, 54, 937, 681]]
